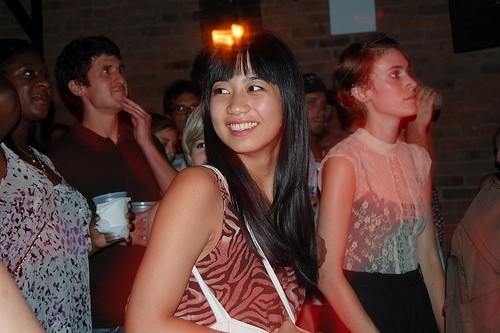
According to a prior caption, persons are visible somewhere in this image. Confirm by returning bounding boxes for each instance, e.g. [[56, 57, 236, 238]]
[[399, 84, 500, 333], [124, 31, 316, 333], [317, 35, 446, 333], [1, 37, 135, 333], [150, 74, 353, 169], [51, 35, 178, 333]]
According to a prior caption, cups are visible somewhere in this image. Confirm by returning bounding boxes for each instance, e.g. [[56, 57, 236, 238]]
[[92, 192, 128, 242], [433, 92, 443, 110], [131, 202, 157, 240]]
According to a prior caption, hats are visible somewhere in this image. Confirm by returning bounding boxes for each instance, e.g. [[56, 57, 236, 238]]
[[302, 71, 325, 93]]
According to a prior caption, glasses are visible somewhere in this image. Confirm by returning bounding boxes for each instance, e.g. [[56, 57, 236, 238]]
[[172, 105, 198, 112]]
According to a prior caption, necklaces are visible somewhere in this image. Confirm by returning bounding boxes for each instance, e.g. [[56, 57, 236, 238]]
[[14, 144, 48, 177]]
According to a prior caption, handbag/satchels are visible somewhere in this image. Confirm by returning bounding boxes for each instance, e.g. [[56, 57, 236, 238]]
[[189, 164, 296, 332]]
